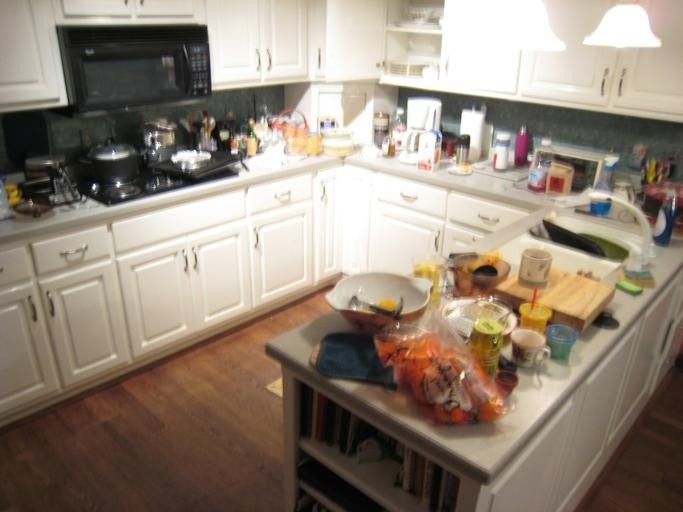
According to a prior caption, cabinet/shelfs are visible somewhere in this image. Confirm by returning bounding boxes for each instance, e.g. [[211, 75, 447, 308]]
[[382, 1, 574, 110], [362, 170, 529, 276], [248, 172, 343, 317], [0, 223, 131, 426], [1, 0, 309, 111], [111, 190, 252, 367]]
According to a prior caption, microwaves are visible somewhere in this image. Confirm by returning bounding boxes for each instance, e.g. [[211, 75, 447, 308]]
[[528, 146, 614, 196], [54, 23, 211, 120]]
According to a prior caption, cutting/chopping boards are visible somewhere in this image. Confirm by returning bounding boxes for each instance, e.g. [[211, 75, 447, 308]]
[[493, 266, 618, 335], [469, 160, 529, 183]]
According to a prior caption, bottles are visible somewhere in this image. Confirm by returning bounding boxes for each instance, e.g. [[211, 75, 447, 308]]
[[653, 187, 678, 249], [374, 112, 388, 149], [413, 263, 439, 312], [526, 138, 553, 193], [491, 133, 511, 171], [454, 134, 472, 166], [247, 127, 258, 156], [391, 108, 406, 152], [460, 319, 503, 379], [513, 124, 529, 167]]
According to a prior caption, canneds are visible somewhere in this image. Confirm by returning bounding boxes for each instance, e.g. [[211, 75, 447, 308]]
[[469, 319, 505, 379], [374, 113, 390, 146]]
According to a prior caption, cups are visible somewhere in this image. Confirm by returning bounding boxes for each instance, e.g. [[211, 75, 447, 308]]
[[509, 329, 552, 373], [520, 302, 553, 335], [612, 181, 635, 210], [546, 325, 578, 362]]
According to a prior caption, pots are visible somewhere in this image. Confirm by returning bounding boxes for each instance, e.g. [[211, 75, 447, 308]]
[[77, 136, 148, 186], [139, 116, 176, 164]]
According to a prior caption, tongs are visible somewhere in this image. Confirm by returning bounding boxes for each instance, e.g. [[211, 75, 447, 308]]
[[348, 294, 404, 323]]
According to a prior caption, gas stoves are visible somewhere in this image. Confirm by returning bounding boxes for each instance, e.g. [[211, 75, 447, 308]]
[[75, 165, 235, 209]]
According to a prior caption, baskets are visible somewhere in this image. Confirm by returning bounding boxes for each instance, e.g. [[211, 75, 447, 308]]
[[270, 109, 308, 137]]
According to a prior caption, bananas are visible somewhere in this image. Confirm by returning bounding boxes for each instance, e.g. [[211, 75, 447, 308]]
[[3, 184, 22, 206]]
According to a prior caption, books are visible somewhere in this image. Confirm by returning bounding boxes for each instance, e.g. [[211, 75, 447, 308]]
[[296, 381, 461, 511]]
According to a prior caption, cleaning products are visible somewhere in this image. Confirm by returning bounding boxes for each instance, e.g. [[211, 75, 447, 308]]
[[653, 188, 676, 246], [590, 154, 619, 213]]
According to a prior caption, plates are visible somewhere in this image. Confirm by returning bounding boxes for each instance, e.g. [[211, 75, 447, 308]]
[[448, 167, 471, 175], [392, 63, 427, 75]]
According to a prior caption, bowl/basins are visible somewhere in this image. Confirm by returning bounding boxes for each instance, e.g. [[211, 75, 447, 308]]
[[449, 256, 512, 296], [440, 298, 516, 343], [324, 270, 433, 333]]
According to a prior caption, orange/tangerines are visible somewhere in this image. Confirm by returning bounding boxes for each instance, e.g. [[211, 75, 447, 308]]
[[272, 119, 322, 156]]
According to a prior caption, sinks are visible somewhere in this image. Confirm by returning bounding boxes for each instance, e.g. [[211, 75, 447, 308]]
[[527, 214, 652, 265]]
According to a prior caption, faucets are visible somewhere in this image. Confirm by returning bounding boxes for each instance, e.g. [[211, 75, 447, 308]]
[[587, 191, 653, 249]]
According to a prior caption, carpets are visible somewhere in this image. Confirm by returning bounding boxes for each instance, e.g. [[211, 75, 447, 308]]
[[266, 376, 283, 397]]
